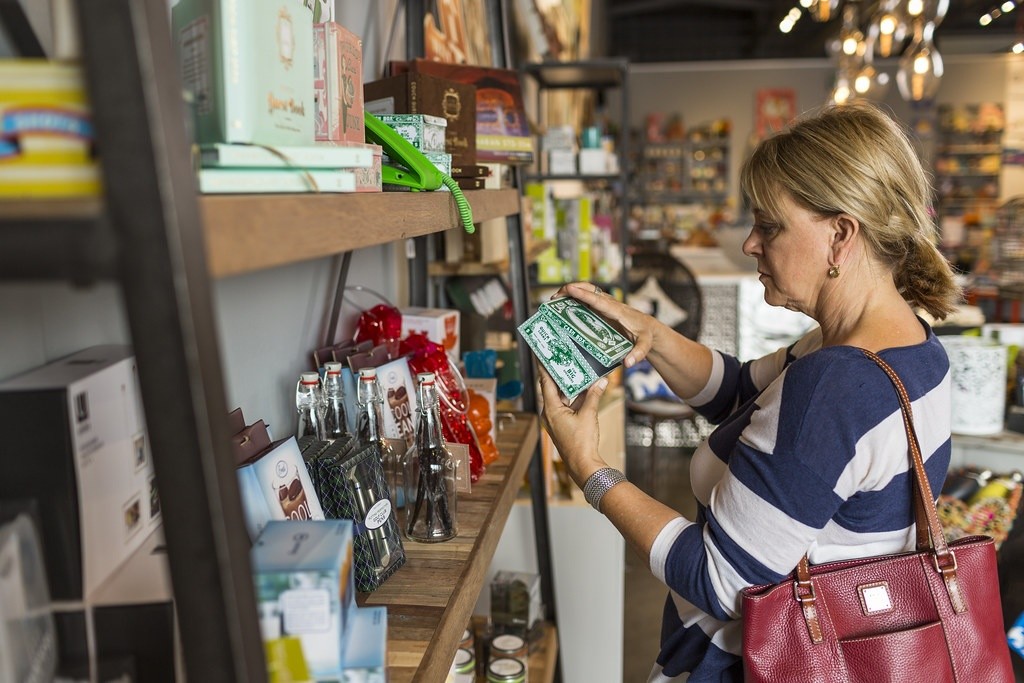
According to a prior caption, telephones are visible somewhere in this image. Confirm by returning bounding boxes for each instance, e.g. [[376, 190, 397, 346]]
[[364, 109, 476, 234]]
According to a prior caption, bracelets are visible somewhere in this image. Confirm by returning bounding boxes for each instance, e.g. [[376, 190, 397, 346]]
[[583, 467, 628, 512]]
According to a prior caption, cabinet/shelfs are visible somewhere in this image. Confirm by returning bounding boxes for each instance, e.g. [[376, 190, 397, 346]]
[[0, 0, 1024, 683]]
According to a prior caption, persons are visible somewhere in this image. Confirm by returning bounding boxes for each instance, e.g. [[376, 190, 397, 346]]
[[534, 98, 953, 683]]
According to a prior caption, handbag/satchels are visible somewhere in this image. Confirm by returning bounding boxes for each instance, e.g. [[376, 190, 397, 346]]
[[742, 347, 1016, 683]]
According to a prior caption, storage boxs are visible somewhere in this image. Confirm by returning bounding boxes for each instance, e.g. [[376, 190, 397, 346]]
[[382, 154, 452, 192], [235, 435, 325, 546], [399, 305, 459, 367], [8, 601, 96, 683], [517, 298, 634, 401], [460, 378, 497, 446], [0, 342, 165, 605], [549, 149, 579, 175], [91, 523, 186, 683], [580, 148, 609, 174], [250, 520, 357, 683], [375, 114, 448, 155]]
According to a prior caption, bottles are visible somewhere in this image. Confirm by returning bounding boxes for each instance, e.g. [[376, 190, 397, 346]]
[[298, 363, 459, 543], [448, 630, 528, 682]]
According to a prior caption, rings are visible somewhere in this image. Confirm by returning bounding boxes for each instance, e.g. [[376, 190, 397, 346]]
[[595, 286, 603, 294]]
